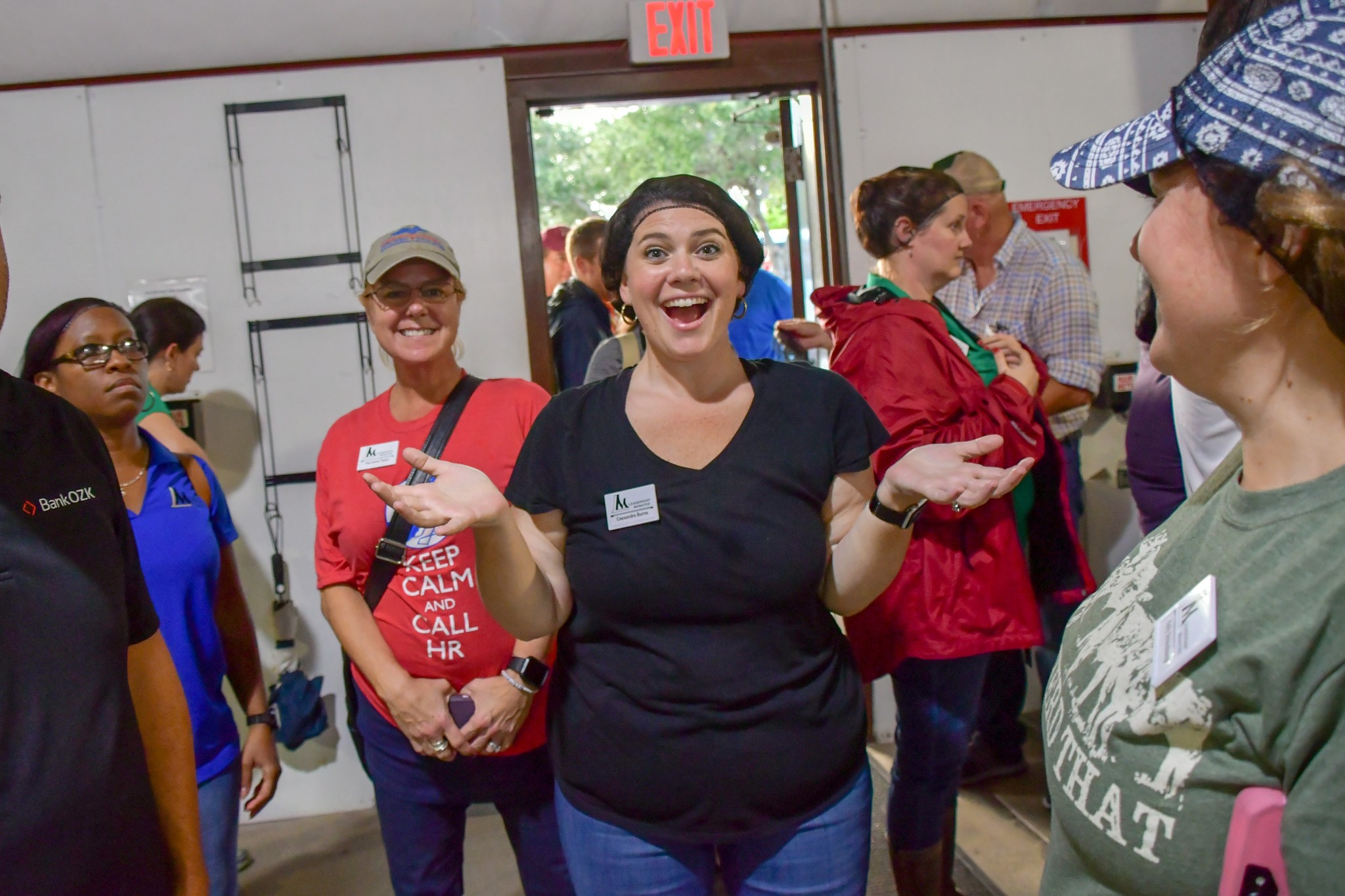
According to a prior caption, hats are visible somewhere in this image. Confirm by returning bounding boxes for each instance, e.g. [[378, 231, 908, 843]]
[[1049, 0, 1345, 195], [366, 225, 460, 285], [541, 226, 572, 250], [933, 151, 1005, 194]]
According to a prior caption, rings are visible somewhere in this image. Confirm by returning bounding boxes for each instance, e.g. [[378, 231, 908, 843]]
[[490, 740, 501, 753], [951, 500, 963, 513], [432, 740, 449, 752]]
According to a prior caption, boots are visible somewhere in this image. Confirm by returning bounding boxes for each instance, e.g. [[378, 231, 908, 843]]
[[888, 807, 973, 896]]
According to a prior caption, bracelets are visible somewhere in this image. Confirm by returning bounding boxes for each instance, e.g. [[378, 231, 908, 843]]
[[500, 668, 540, 694]]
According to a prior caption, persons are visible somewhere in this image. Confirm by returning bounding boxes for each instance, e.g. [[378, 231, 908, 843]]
[[0, 234, 283, 896], [1124, 0, 1295, 539], [314, 223, 577, 895], [361, 175, 1035, 896], [540, 217, 793, 395], [1038, 0, 1344, 896]]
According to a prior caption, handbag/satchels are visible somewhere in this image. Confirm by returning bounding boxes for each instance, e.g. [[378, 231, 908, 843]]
[[341, 647, 373, 780]]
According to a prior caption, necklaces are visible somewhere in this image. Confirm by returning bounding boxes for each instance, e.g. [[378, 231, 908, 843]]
[[119, 448, 150, 496]]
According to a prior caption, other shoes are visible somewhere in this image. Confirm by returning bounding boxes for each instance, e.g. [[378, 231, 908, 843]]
[[961, 731, 1028, 786], [237, 845, 252, 870]]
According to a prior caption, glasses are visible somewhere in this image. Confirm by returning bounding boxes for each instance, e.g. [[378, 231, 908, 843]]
[[364, 278, 463, 307], [48, 338, 149, 369]]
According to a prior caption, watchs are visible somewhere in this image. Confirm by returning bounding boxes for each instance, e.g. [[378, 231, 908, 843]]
[[507, 656, 550, 689], [869, 485, 928, 530], [246, 712, 280, 733]]
